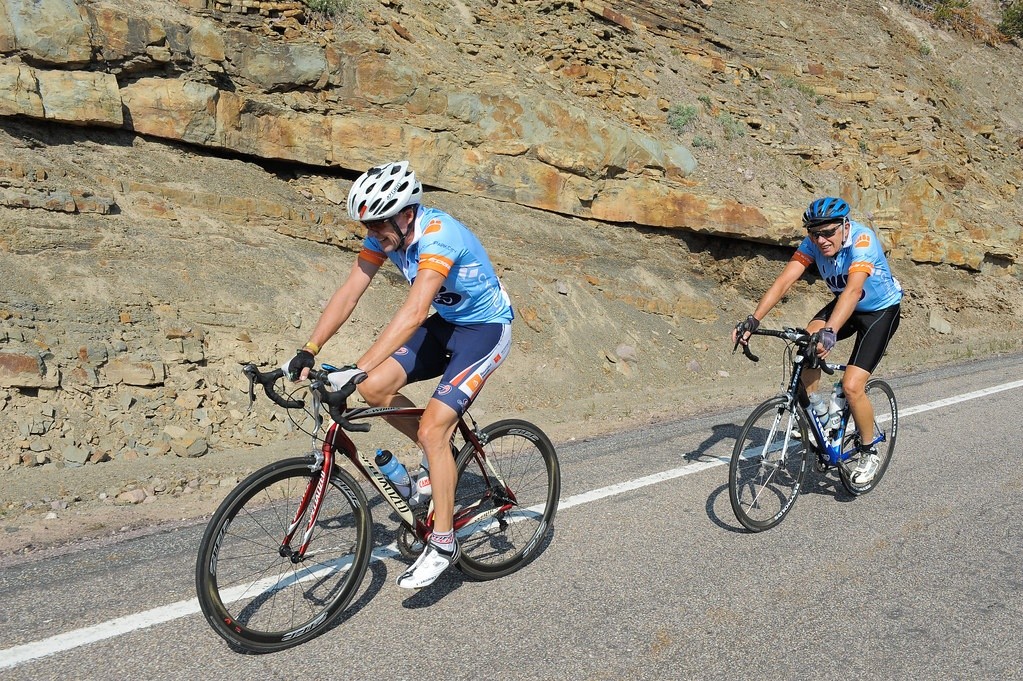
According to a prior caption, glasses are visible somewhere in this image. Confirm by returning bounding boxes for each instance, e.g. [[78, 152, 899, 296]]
[[807, 219, 848, 239], [360, 205, 416, 231]]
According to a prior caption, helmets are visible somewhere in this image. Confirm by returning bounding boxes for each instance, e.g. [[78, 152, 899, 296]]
[[801, 197, 851, 229], [346, 161, 423, 222]]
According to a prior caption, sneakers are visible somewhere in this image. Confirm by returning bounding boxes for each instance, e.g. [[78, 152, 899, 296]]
[[791, 424, 811, 437], [849, 448, 880, 483], [395, 534, 461, 589], [408, 492, 431, 506]]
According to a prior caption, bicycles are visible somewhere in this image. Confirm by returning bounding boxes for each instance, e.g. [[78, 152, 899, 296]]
[[730, 328, 898, 532], [194, 365, 562, 653]]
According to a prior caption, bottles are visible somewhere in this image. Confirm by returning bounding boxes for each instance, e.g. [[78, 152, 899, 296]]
[[372, 448, 418, 500], [416, 451, 432, 496], [827, 382, 846, 430], [809, 391, 831, 430]]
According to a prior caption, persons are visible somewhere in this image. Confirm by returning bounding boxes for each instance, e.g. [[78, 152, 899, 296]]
[[731, 197, 904, 485], [279, 160, 516, 589]]
